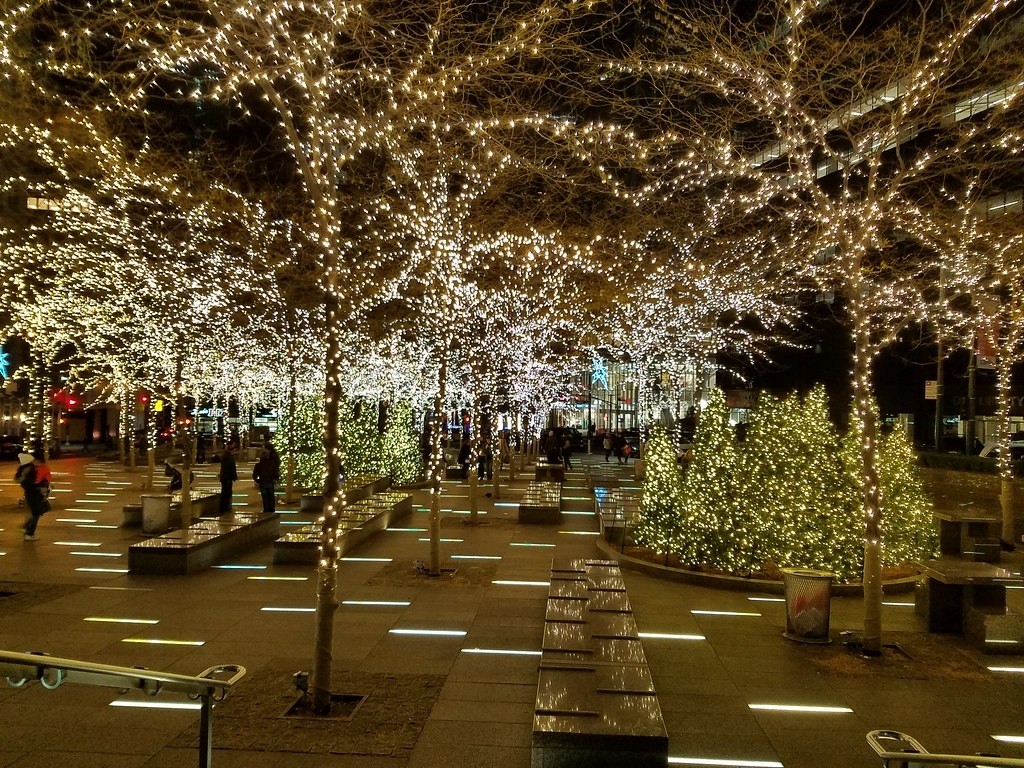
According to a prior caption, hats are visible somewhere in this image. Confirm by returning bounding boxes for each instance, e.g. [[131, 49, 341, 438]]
[[18, 453, 35, 465]]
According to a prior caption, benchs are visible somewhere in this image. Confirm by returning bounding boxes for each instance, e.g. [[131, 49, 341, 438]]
[[585, 464, 619, 492], [530, 556, 669, 768], [535, 456, 565, 481], [301, 473, 393, 512], [122, 489, 222, 526], [593, 485, 642, 537], [274, 491, 413, 563], [519, 480, 562, 525], [128, 509, 282, 576]]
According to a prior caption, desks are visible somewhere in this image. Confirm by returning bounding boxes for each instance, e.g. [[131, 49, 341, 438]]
[[910, 562, 1024, 634], [932, 507, 1001, 556]]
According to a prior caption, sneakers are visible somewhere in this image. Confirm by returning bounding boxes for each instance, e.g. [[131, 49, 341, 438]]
[[18, 527, 26, 533], [24, 534, 40, 541]]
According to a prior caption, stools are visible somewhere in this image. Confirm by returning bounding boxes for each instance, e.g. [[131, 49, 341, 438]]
[[973, 537, 1001, 562], [914, 576, 923, 615], [969, 607, 1024, 654]]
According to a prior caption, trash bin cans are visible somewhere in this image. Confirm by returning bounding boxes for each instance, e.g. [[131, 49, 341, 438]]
[[779, 568, 836, 646], [140, 494, 174, 536]]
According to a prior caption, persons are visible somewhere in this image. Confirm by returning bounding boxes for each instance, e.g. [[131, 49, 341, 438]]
[[603, 433, 631, 464], [263, 443, 280, 466], [458, 432, 509, 483], [196, 433, 206, 463], [252, 450, 279, 512], [543, 429, 573, 472], [163, 442, 194, 490], [34, 453, 51, 498], [13, 453, 51, 541], [218, 441, 237, 514], [339, 458, 345, 489]]
